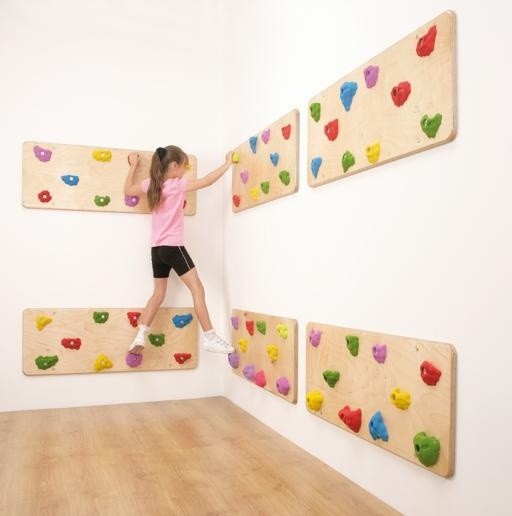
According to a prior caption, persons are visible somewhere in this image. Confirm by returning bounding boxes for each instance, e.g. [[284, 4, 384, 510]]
[[123, 146, 236, 355]]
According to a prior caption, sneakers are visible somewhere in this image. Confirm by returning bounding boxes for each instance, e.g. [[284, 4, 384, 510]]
[[127, 332, 146, 355]]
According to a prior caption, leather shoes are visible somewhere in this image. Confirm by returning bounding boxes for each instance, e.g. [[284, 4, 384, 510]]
[[203, 334, 236, 354]]
